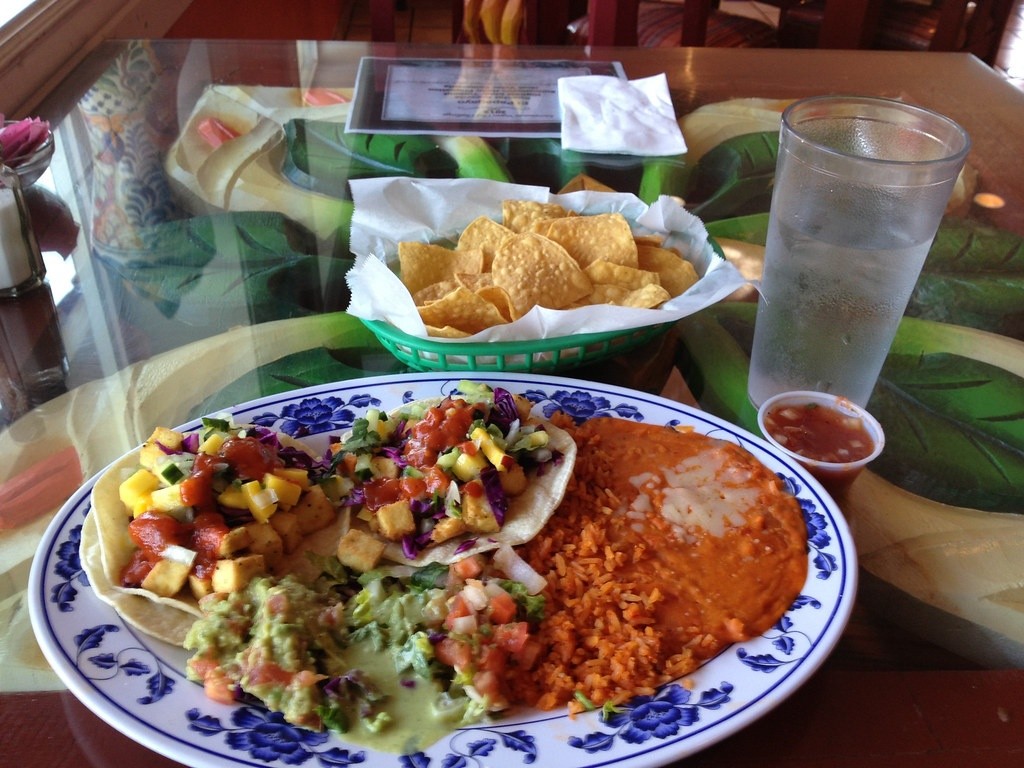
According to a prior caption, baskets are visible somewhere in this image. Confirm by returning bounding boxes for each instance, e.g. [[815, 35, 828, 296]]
[[361, 233, 723, 371]]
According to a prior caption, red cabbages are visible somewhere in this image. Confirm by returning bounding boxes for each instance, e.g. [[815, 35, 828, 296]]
[[152, 385, 568, 564]]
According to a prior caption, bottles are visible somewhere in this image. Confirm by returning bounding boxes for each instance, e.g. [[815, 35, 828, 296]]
[[0, 155, 47, 300], [0, 282, 70, 443]]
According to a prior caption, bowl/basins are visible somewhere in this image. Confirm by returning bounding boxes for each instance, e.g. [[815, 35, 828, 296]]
[[756, 390, 885, 504], [5, 121, 55, 191]]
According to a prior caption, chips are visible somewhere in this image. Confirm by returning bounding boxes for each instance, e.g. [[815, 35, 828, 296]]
[[397, 195, 700, 342]]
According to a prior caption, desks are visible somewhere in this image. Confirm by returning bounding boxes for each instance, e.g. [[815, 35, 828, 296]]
[[1, 40, 1024, 768]]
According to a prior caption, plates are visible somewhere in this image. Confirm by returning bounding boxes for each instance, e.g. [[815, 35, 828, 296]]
[[26, 370, 858, 768]]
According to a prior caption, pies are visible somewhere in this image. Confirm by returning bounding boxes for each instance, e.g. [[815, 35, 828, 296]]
[[75, 422, 349, 649], [342, 396, 578, 568]]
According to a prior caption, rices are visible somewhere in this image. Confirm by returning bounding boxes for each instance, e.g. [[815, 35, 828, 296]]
[[524, 410, 746, 722]]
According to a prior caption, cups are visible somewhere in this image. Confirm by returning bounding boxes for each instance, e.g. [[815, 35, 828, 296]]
[[746, 93, 970, 412]]
